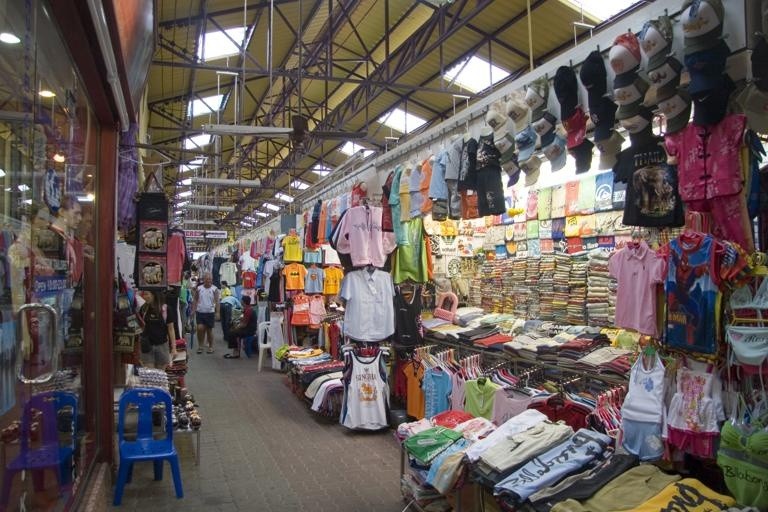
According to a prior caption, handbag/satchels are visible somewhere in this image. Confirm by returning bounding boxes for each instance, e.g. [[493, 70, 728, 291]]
[[113, 305, 147, 355], [65, 326, 86, 348]]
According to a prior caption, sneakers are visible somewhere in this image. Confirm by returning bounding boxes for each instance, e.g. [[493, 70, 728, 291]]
[[197, 347, 240, 359]]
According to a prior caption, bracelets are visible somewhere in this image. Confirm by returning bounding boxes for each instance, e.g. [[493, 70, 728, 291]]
[[170, 343, 177, 346]]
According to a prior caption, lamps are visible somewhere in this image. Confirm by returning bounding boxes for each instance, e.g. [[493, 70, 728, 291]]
[[182, 0, 296, 226]]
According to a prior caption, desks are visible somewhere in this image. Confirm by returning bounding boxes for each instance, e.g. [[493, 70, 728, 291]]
[[123, 422, 202, 470], [395, 432, 552, 512]]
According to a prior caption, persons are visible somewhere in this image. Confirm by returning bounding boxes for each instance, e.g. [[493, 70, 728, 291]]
[[190, 271, 221, 355], [6, 198, 53, 405], [44, 192, 84, 284], [221, 295, 255, 359], [137, 285, 178, 370], [218, 280, 230, 299], [218, 295, 242, 342]]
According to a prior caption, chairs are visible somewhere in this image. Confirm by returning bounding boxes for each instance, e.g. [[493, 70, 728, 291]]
[[0, 390, 80, 512], [242, 306, 269, 357], [114, 387, 182, 505], [257, 321, 286, 371]]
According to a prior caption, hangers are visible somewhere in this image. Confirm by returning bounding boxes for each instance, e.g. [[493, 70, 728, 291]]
[[117, 134, 171, 166], [633, 339, 767, 421], [625, 211, 767, 324], [340, 340, 625, 437]]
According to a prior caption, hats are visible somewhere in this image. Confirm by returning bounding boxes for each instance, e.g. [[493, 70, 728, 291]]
[[642, 56, 683, 107], [593, 128, 625, 171], [680, 0, 725, 47], [735, 78, 768, 134], [579, 50, 610, 110], [485, 64, 594, 188], [607, 32, 644, 89], [618, 104, 654, 153], [612, 73, 649, 120], [639, 14, 676, 74], [750, 36, 768, 84], [683, 39, 732, 69], [655, 88, 693, 135], [589, 96, 618, 143], [685, 67, 738, 128]]
